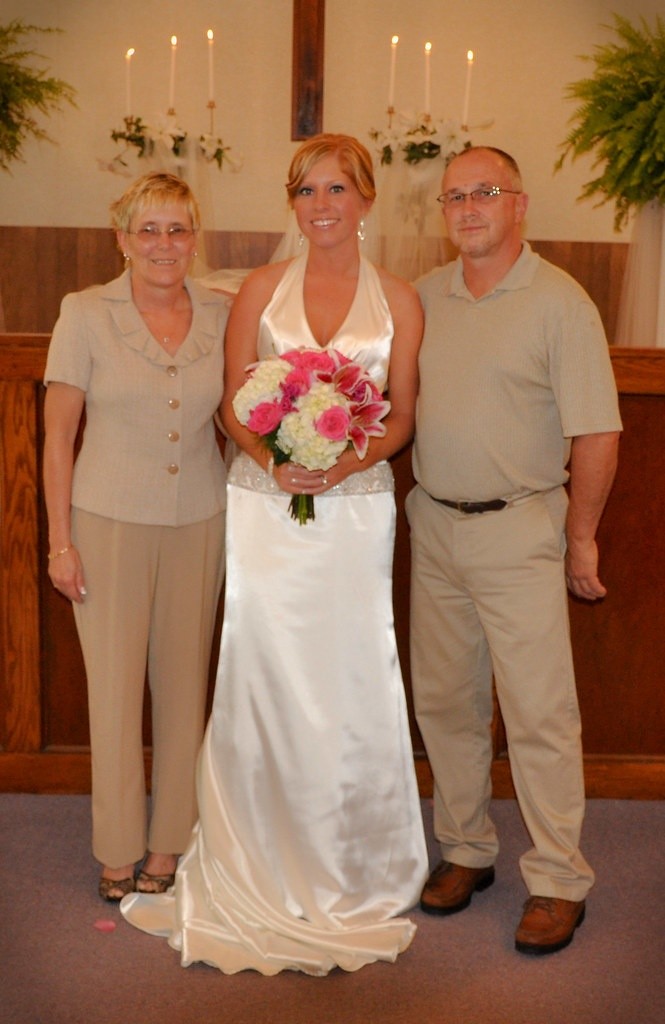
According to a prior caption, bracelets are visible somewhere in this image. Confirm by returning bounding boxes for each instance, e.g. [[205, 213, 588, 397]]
[[47, 544, 74, 560], [268, 457, 274, 477]]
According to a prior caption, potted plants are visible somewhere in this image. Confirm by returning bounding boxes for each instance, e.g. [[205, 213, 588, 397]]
[[462, 50, 474, 127]]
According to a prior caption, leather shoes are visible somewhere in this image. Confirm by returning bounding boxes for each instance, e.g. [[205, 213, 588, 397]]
[[513, 896, 587, 956], [419, 859, 497, 917]]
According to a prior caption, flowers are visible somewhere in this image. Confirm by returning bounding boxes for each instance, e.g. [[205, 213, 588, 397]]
[[93, 118, 245, 184], [353, 114, 503, 184], [231, 346, 391, 527]]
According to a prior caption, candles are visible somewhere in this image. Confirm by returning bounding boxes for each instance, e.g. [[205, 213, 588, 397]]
[[424, 43, 432, 115], [169, 36, 177, 110], [126, 49, 134, 120], [206, 29, 215, 102], [388, 36, 399, 111]]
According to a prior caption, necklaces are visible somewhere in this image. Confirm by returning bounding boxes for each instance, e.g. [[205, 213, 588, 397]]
[[145, 314, 182, 342]]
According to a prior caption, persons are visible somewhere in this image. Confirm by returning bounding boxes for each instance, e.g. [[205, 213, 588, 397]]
[[209, 145, 624, 956], [42, 173, 233, 902], [119, 134, 430, 977]]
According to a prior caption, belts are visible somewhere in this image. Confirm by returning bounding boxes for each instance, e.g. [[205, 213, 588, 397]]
[[427, 493, 507, 515]]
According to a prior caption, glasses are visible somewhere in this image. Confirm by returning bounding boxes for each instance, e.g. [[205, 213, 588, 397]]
[[435, 185, 522, 208], [124, 224, 195, 243]]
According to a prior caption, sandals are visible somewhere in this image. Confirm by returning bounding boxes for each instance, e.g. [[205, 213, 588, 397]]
[[98, 868, 137, 903], [135, 868, 175, 894]]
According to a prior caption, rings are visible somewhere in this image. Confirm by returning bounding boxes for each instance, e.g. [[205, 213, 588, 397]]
[[320, 475, 328, 485]]
